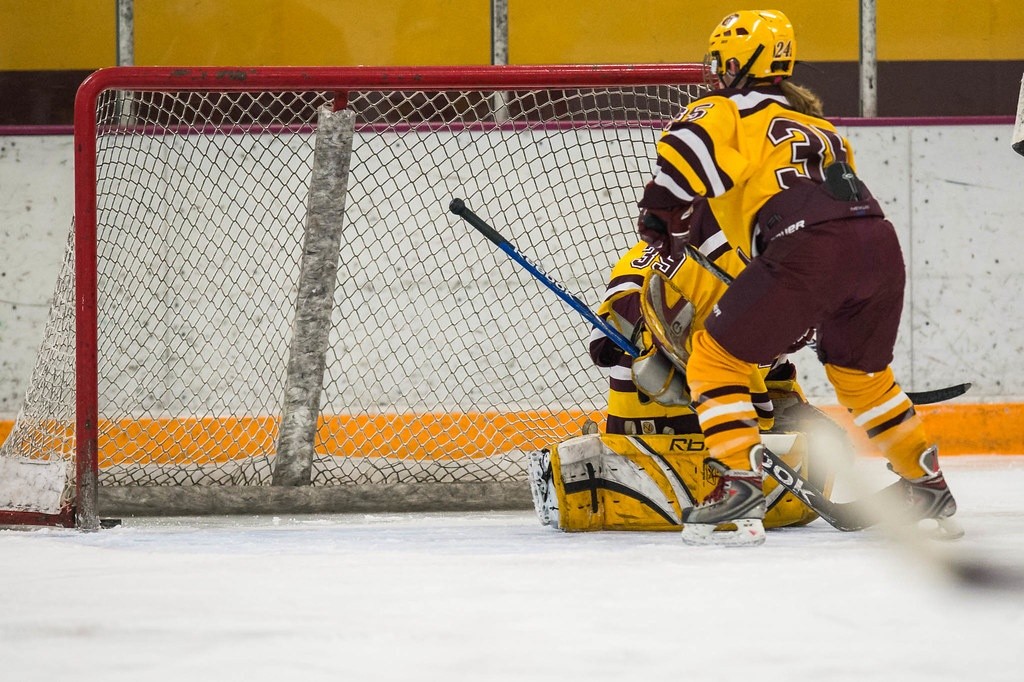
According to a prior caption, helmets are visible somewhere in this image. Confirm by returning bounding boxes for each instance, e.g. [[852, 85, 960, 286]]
[[703, 9, 796, 91]]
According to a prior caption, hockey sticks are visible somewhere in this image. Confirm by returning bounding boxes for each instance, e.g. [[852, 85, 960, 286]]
[[640, 211, 976, 410], [448, 195, 915, 534]]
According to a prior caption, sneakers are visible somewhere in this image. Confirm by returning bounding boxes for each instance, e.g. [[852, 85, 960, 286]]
[[681, 458, 765, 547], [867, 445, 965, 539]]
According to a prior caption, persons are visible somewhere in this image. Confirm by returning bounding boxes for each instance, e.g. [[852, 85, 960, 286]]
[[529, 203, 854, 533], [637, 8, 965, 544]]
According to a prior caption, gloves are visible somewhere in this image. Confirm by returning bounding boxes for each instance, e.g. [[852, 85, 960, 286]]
[[638, 205, 691, 260]]
